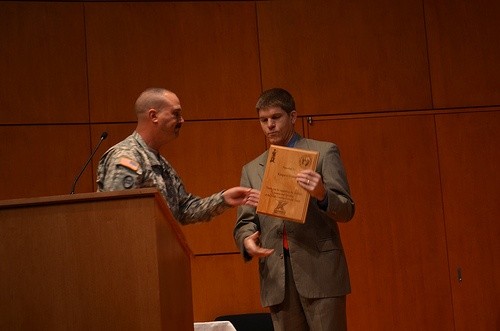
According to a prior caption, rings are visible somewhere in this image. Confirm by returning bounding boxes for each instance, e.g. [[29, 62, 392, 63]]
[[307, 179, 311, 185]]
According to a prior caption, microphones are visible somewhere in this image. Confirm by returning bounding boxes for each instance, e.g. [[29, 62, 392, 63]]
[[72, 132, 108, 194]]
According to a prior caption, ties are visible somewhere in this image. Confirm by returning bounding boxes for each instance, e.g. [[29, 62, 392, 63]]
[[283, 225, 289, 249]]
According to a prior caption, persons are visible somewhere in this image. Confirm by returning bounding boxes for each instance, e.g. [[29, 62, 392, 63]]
[[232, 88, 356, 331], [96, 86, 260, 224]]
